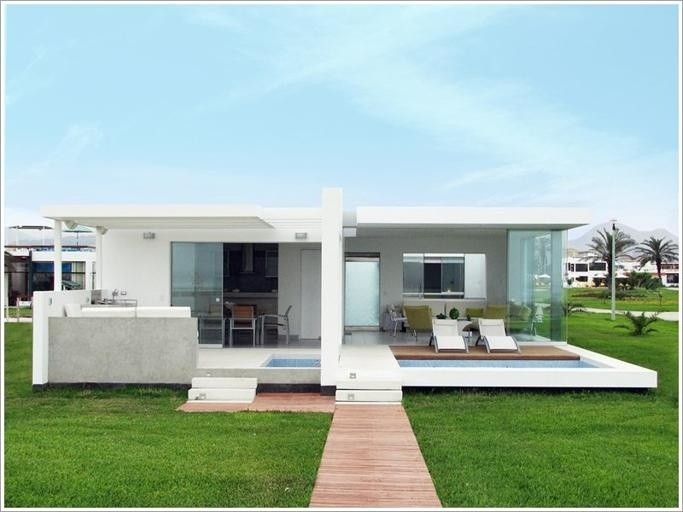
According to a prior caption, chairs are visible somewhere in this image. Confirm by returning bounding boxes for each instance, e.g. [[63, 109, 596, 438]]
[[200, 300, 294, 351], [385, 301, 538, 358]]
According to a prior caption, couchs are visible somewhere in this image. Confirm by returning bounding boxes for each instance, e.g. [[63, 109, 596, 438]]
[[31, 288, 199, 388]]
